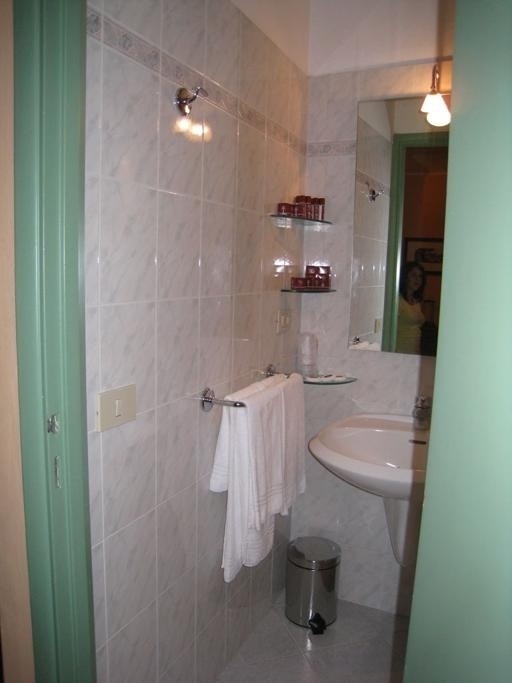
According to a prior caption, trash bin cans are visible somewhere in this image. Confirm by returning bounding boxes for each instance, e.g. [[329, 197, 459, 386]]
[[286, 534, 341, 634]]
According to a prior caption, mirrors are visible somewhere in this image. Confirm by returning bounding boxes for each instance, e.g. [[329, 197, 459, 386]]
[[348, 98, 452, 358]]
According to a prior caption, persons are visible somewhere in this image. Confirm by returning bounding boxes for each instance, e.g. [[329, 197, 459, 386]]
[[396, 262, 429, 353]]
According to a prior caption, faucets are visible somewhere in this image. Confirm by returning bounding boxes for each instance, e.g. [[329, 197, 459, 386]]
[[412, 395, 431, 430]]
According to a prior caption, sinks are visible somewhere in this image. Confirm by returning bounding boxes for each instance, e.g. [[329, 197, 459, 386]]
[[320, 430, 429, 471]]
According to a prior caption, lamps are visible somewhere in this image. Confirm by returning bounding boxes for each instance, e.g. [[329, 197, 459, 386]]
[[420, 65, 452, 129]]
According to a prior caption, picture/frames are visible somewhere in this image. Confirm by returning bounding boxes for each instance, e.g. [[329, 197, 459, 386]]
[[404, 238, 444, 303]]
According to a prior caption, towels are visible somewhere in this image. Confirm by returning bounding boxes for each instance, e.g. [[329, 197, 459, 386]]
[[210, 374, 286, 493], [220, 372, 308, 582]]
